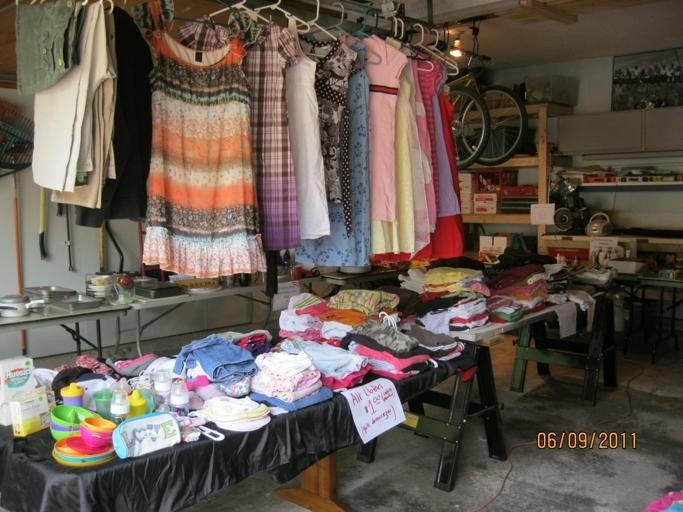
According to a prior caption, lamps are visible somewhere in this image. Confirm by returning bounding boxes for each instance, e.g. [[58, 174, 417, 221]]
[[449, 33, 463, 58]]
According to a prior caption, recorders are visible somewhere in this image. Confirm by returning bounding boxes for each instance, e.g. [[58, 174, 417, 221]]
[[584, 212, 613, 236]]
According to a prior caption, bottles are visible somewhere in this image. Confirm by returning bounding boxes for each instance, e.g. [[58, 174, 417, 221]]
[[219, 249, 320, 290], [110, 368, 189, 425]]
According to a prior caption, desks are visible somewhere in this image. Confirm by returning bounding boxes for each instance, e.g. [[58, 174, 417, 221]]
[[321, 260, 408, 299], [612, 270, 654, 355], [637, 274, 683, 364], [407, 287, 622, 462], [114, 272, 324, 358], [0, 295, 132, 360], [0, 343, 478, 510]]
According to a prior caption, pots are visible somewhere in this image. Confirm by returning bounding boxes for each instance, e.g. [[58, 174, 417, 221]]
[[0, 295, 46, 317]]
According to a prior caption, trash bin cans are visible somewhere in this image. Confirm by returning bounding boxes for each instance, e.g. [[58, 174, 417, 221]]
[[623, 297, 658, 354]]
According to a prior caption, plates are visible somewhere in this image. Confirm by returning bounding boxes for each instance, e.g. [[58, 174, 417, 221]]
[[87, 284, 114, 297], [52, 439, 115, 467], [188, 285, 223, 294]]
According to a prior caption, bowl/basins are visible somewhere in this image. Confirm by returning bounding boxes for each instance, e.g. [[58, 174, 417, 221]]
[[91, 275, 112, 286], [49, 404, 117, 447]]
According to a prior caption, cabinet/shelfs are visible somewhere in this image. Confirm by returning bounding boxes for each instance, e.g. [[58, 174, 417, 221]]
[[538, 150, 682, 274], [450, 103, 574, 260]]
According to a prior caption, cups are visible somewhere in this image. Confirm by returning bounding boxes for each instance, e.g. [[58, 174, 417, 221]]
[[93, 388, 112, 420], [60, 382, 84, 408]]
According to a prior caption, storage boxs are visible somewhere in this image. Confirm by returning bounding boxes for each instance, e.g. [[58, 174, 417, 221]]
[[473, 193, 497, 215], [457, 174, 478, 215], [479, 234, 512, 258]]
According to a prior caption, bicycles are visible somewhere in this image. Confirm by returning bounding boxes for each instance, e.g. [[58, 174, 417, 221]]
[[446, 48, 527, 168]]
